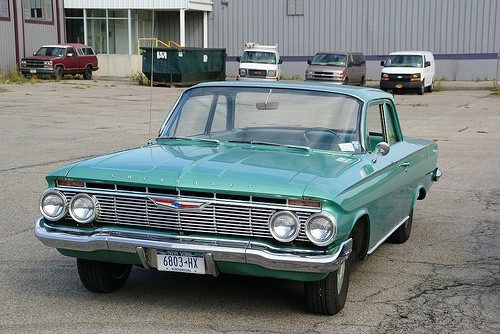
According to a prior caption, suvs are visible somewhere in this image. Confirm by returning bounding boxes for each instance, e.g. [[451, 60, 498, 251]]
[[20, 44, 98, 80]]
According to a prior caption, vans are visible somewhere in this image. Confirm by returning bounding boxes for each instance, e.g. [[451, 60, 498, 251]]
[[380, 51, 436, 95], [236, 42, 283, 81], [305, 52, 367, 87]]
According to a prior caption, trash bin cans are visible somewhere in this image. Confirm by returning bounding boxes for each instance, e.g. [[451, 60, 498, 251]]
[[138, 45, 184, 87], [174, 47, 227, 87]]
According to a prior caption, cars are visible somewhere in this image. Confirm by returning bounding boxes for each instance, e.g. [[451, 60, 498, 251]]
[[35, 80, 441, 316]]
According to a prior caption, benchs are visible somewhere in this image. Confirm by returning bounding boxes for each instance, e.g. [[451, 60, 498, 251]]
[[237, 129, 383, 154]]
